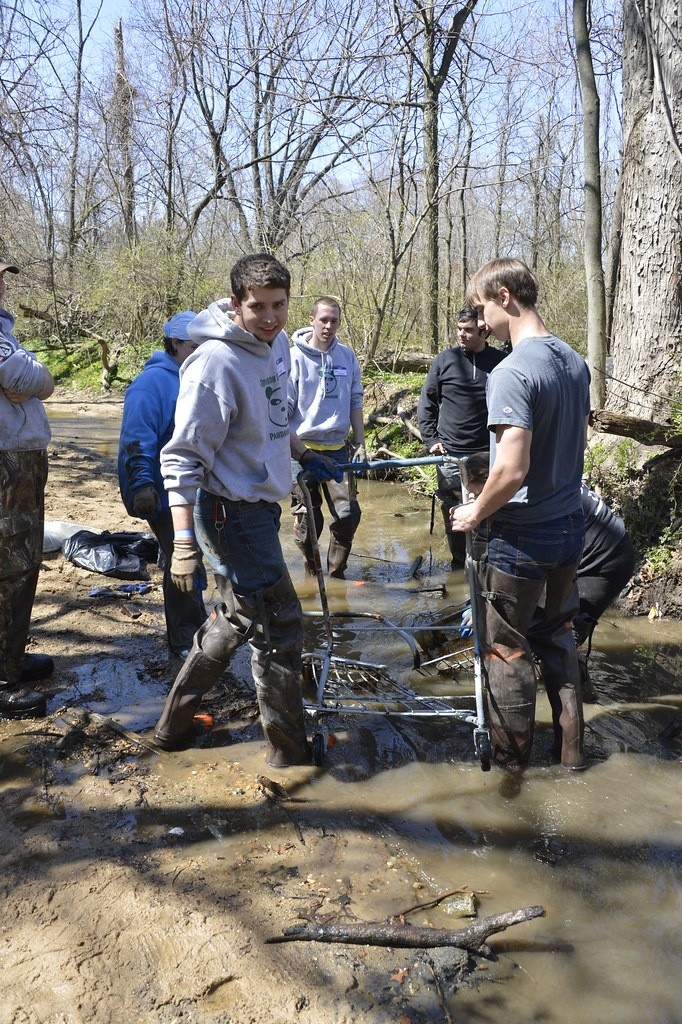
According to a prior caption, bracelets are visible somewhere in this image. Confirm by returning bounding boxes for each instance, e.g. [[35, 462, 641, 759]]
[[174, 529, 193, 538]]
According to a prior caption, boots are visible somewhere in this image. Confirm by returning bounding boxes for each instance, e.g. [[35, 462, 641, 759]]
[[533, 560, 587, 773], [232, 563, 317, 768], [324, 472, 362, 579], [436, 488, 465, 570], [476, 563, 548, 765], [290, 482, 324, 575], [571, 614, 602, 704], [153, 573, 248, 749]]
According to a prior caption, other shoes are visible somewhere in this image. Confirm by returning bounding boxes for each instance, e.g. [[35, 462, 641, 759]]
[[0, 681, 46, 719], [21, 652, 54, 681]]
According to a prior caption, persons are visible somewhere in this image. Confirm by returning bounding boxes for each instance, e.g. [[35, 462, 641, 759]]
[[447, 256, 594, 772], [0, 305, 55, 720], [458, 451, 634, 653], [283, 294, 365, 578], [143, 253, 335, 768], [415, 310, 508, 571], [117, 309, 210, 663]]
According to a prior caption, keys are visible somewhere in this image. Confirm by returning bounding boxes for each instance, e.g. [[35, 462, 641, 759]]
[[214, 521, 224, 545]]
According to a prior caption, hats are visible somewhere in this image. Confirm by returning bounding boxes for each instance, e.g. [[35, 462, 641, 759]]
[[163, 310, 197, 340], [0, 259, 20, 275]]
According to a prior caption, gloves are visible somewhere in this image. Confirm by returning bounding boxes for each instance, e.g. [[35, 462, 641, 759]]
[[170, 531, 208, 601], [458, 608, 474, 638], [299, 448, 344, 484], [352, 443, 367, 474], [133, 487, 162, 521]]
